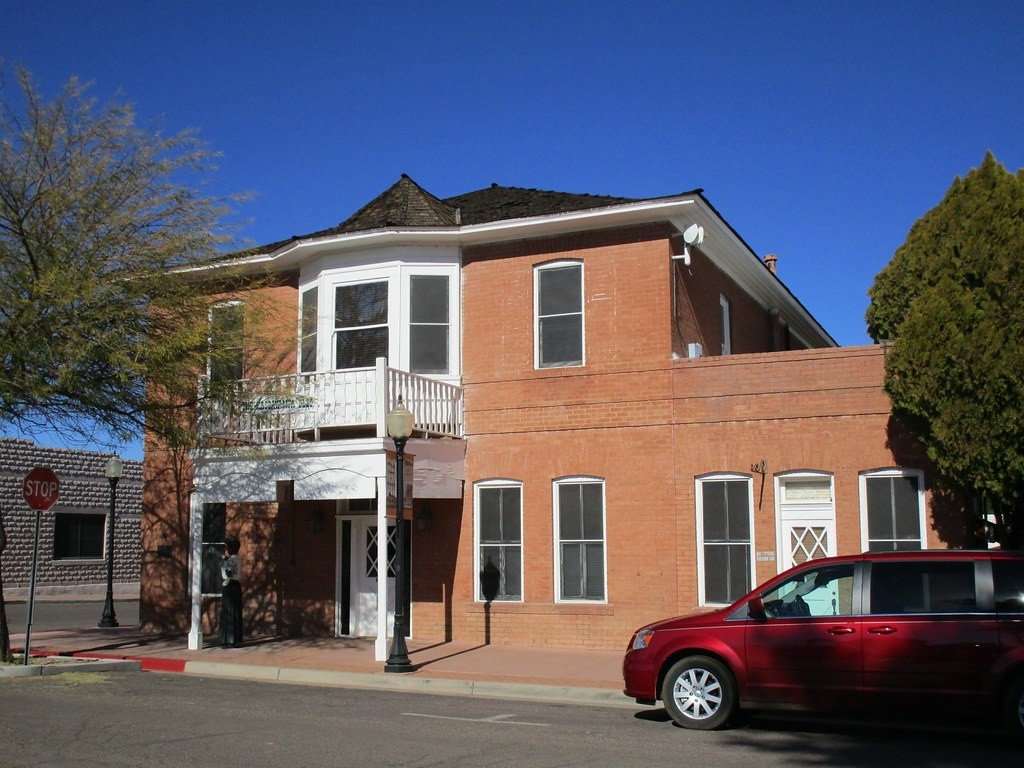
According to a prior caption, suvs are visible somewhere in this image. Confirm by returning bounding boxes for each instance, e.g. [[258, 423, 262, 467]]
[[623, 549, 1024, 748]]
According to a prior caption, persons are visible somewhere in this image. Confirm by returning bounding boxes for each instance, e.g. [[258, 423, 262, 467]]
[[207, 535, 243, 649]]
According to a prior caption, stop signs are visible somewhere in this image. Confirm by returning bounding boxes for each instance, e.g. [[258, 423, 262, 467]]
[[23, 466, 61, 511]]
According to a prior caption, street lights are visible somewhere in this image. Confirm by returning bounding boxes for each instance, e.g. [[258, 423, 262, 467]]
[[98, 450, 124, 627], [384, 393, 414, 675]]
